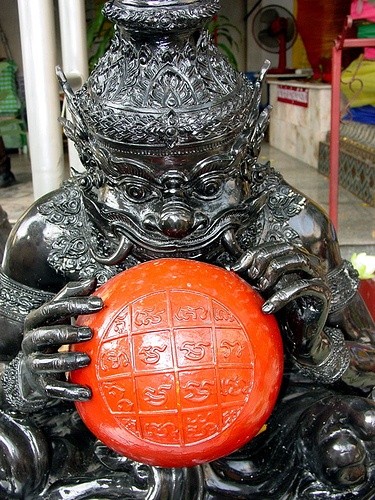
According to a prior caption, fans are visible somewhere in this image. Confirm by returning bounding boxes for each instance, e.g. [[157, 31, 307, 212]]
[[252, 4, 301, 76]]
